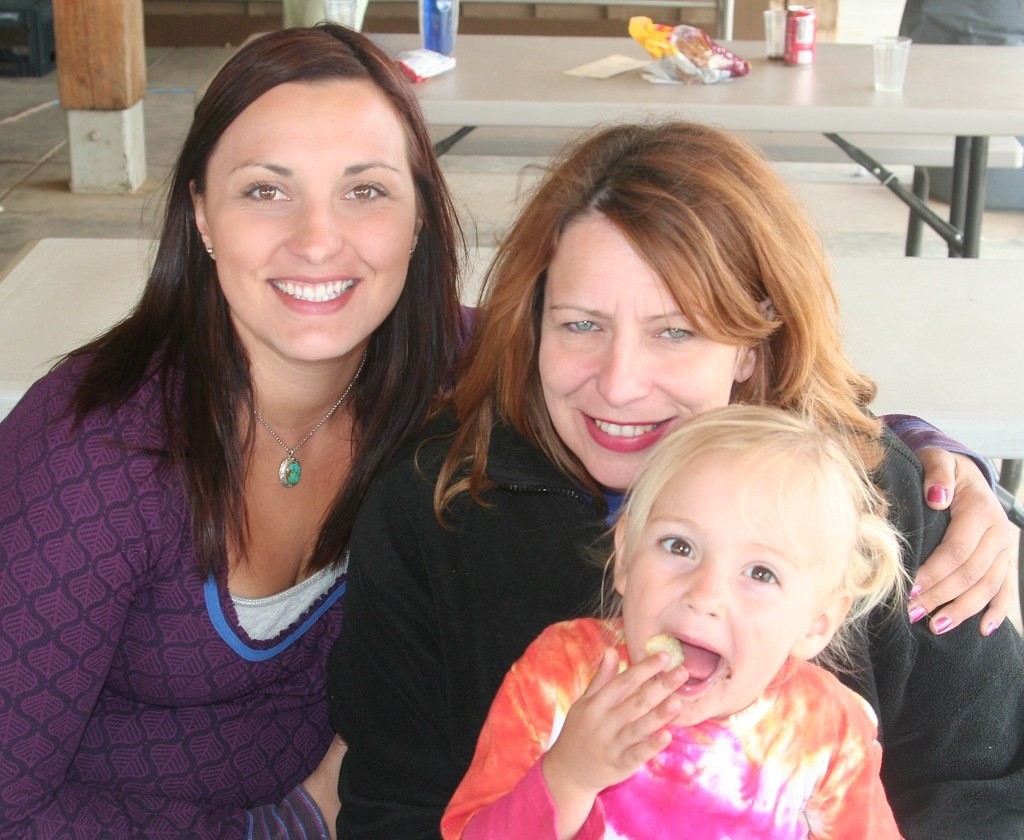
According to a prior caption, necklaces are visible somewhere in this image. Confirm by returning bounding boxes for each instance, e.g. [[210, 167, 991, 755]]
[[232, 350, 370, 487]]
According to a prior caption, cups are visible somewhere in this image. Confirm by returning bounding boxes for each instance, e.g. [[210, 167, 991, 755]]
[[423, 0, 453, 56], [763, 11, 789, 57], [873, 34, 913, 93]]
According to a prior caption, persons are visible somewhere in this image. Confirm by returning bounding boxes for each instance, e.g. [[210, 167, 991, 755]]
[[327, 121, 1024, 840], [0, 26, 1024, 840], [441, 405, 906, 840]]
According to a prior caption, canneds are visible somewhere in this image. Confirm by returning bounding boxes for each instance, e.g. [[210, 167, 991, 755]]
[[784, 5, 817, 67]]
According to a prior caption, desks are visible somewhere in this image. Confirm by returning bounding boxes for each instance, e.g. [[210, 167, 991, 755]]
[[0, 238, 1024, 495], [193, 34, 1023, 260]]
